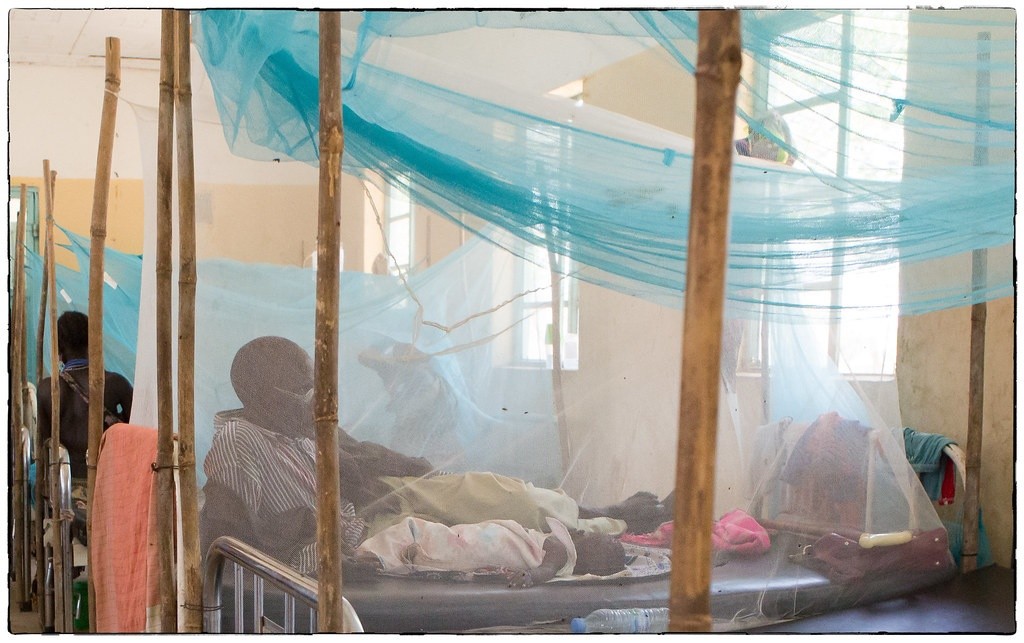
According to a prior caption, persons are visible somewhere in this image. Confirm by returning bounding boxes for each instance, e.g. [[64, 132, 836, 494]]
[[355, 514, 625, 588], [198, 335, 666, 568], [36, 311, 134, 478], [347, 328, 459, 461]]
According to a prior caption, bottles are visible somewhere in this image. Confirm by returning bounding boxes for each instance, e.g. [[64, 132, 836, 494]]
[[570, 608, 670, 633]]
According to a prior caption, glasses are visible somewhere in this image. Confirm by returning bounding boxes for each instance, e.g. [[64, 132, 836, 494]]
[[273, 386, 315, 404]]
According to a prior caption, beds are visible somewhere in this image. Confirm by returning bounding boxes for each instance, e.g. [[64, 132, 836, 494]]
[[13, 379, 88, 634], [201, 423, 1014, 634]]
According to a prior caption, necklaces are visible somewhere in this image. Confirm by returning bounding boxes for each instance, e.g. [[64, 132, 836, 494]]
[[63, 359, 88, 370]]
